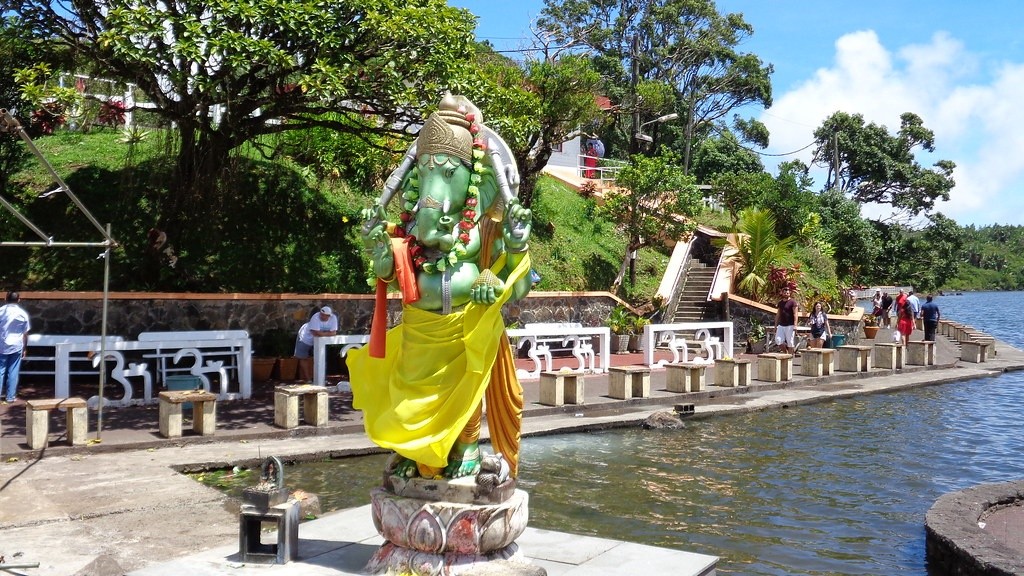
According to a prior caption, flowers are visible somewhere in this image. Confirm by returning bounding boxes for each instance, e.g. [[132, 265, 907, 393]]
[[864, 314, 879, 327], [834, 326, 845, 335]]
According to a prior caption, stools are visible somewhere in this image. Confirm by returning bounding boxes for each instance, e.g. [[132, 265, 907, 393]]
[[756, 352, 794, 382], [607, 365, 651, 400], [662, 363, 708, 393], [274, 384, 329, 429], [875, 315, 996, 370], [158, 390, 217, 439], [798, 348, 837, 377], [538, 371, 585, 407], [25, 397, 88, 450], [836, 344, 873, 372], [713, 359, 752, 388]]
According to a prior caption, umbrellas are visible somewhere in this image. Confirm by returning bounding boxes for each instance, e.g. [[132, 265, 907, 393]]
[[586, 139, 605, 157]]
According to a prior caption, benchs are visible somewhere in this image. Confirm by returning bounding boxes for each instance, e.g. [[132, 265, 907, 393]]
[[138, 328, 255, 388], [524, 323, 592, 360], [18, 334, 125, 385]]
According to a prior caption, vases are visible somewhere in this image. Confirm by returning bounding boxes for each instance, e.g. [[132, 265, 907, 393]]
[[863, 326, 880, 339], [832, 335, 847, 349]]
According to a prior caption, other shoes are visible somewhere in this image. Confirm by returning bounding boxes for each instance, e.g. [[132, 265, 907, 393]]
[[887, 324, 891, 329]]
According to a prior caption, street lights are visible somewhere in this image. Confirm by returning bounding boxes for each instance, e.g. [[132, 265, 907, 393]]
[[631, 112, 680, 285]]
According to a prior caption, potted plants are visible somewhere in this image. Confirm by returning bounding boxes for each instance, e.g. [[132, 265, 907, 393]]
[[249, 328, 314, 383], [506, 320, 520, 359], [628, 316, 652, 354], [604, 301, 635, 355], [747, 315, 767, 354]]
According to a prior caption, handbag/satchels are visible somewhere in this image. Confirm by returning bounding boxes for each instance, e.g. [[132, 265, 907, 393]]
[[894, 327, 901, 342]]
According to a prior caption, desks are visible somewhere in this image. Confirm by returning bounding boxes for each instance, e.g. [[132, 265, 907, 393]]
[[764, 326, 812, 353]]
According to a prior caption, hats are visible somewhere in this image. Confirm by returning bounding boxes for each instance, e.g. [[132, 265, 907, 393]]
[[321, 306, 331, 315]]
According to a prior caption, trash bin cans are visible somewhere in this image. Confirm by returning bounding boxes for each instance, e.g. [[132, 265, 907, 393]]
[[830, 334, 846, 349], [166, 375, 201, 408]]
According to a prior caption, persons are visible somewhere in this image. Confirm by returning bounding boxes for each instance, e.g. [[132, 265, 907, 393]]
[[806, 302, 832, 348], [894, 289, 907, 315], [583, 143, 597, 179], [907, 290, 920, 329], [872, 293, 882, 326], [0, 290, 31, 403], [895, 300, 916, 345], [774, 287, 798, 357], [882, 292, 894, 330], [293, 306, 338, 385], [871, 290, 883, 301], [919, 295, 940, 341]]
